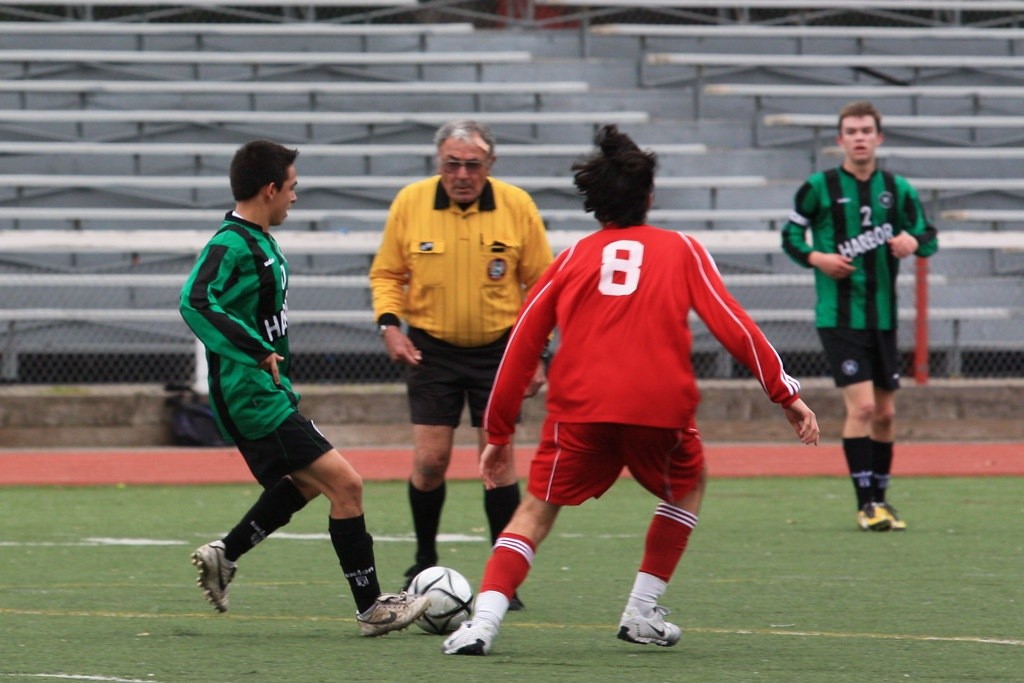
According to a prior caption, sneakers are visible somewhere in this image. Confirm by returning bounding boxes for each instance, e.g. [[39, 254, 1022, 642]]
[[191, 540, 237, 612], [857, 506, 892, 530], [878, 502, 906, 530], [617, 606, 681, 646], [355, 589, 430, 637], [441, 621, 492, 656]]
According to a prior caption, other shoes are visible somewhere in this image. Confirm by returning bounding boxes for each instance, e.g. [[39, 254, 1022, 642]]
[[508, 590, 525, 611], [401, 564, 433, 594]]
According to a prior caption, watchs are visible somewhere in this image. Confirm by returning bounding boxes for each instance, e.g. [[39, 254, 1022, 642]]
[[377, 325, 387, 334]]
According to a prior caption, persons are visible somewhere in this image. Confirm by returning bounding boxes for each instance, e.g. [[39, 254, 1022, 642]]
[[441, 124, 821, 656], [177, 138, 430, 640], [370, 121, 558, 610], [781, 102, 938, 530]]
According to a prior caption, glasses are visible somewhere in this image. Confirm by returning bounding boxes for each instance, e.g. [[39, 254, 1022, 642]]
[[439, 158, 487, 176]]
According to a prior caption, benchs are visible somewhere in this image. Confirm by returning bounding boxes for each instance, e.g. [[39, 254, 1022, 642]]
[[0, 0, 1024, 380]]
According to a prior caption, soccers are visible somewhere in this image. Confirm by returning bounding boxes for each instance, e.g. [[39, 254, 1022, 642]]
[[406, 565, 476, 635]]
